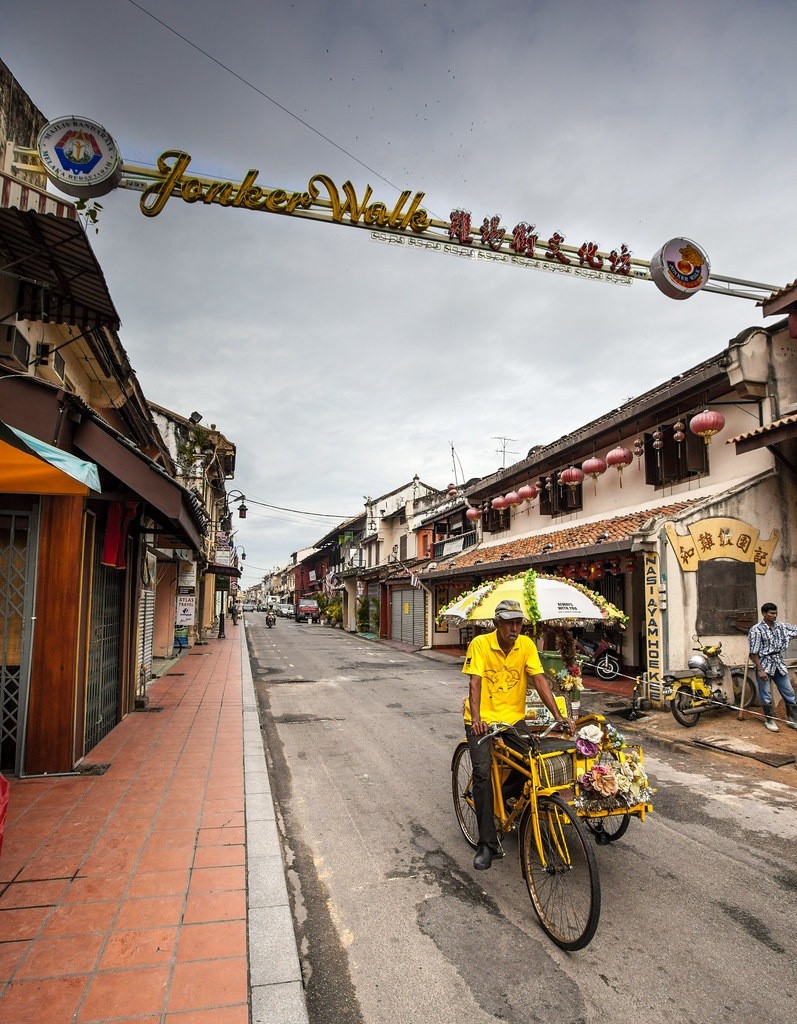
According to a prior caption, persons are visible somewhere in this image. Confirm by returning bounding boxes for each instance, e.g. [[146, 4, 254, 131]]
[[748, 602, 797, 732], [461, 599, 576, 870], [232, 600, 239, 625], [265, 604, 276, 625]]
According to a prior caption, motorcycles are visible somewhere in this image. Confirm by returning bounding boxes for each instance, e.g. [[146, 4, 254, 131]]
[[661, 634, 758, 727]]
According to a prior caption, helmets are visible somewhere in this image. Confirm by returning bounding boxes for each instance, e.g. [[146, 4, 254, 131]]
[[688, 655, 708, 673]]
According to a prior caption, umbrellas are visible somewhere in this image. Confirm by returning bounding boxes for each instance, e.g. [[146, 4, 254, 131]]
[[435, 568, 629, 643]]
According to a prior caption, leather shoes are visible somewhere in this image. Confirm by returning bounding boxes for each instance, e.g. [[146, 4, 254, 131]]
[[474, 845, 495, 870], [505, 802, 523, 824]]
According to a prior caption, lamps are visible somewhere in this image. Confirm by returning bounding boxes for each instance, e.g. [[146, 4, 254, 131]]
[[500, 553, 511, 560], [594, 533, 609, 545], [188, 411, 203, 424], [190, 486, 213, 524], [541, 543, 553, 553], [448, 562, 457, 569], [474, 558, 483, 565], [429, 567, 436, 572]]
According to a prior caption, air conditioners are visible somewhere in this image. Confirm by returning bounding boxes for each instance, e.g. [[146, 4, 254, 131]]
[[0, 324, 32, 372], [388, 554, 396, 563], [37, 343, 66, 385]]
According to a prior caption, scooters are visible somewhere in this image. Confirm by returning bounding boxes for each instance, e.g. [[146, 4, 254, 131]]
[[265, 612, 274, 628], [574, 633, 621, 682]]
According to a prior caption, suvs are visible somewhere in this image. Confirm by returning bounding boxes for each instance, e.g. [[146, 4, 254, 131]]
[[297, 598, 319, 623]]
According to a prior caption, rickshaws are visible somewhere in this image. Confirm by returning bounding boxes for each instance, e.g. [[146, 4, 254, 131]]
[[450, 711, 655, 951]]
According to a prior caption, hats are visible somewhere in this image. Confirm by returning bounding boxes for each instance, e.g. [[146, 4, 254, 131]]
[[495, 599, 525, 620]]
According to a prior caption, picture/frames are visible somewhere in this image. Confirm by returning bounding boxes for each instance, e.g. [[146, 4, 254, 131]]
[[435, 588, 449, 633]]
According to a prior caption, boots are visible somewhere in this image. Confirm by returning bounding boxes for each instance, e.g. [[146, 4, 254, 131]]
[[762, 703, 780, 732], [784, 704, 797, 729]]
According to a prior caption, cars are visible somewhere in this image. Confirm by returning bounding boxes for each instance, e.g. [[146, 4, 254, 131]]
[[243, 603, 254, 613], [287, 605, 296, 619], [257, 603, 291, 617]]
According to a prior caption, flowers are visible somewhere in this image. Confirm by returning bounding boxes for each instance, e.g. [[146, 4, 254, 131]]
[[574, 723, 655, 807], [434, 569, 629, 691]]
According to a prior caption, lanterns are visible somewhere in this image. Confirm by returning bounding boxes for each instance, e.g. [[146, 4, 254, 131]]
[[561, 465, 584, 504], [673, 421, 685, 458], [582, 457, 607, 496], [633, 437, 644, 471], [465, 471, 564, 526], [606, 445, 633, 488], [653, 431, 663, 467], [447, 483, 456, 497], [690, 410, 725, 444]]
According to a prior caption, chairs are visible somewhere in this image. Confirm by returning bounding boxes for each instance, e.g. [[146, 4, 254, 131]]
[[496, 713, 604, 759]]
